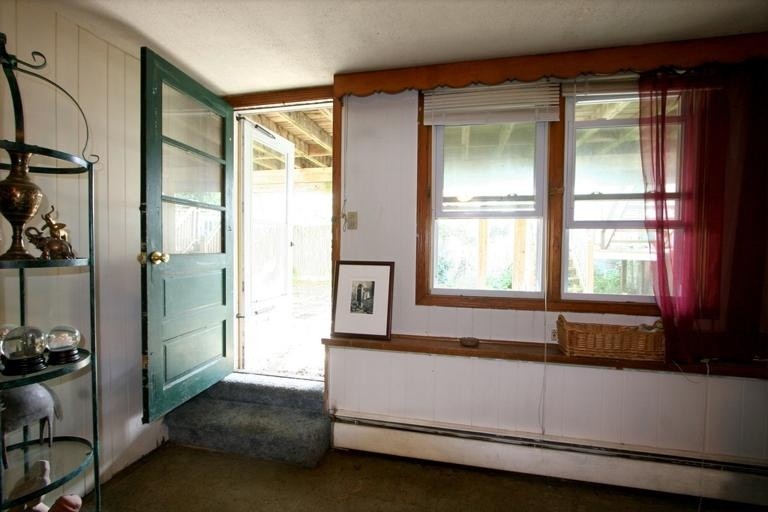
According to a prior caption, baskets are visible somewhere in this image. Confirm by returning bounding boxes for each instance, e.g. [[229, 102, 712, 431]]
[[556, 314, 666, 362]]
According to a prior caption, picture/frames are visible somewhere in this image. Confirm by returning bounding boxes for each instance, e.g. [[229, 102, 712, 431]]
[[330, 260, 395, 341]]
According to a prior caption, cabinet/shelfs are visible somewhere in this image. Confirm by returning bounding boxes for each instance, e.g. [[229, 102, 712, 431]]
[[0, 32, 102, 512]]
